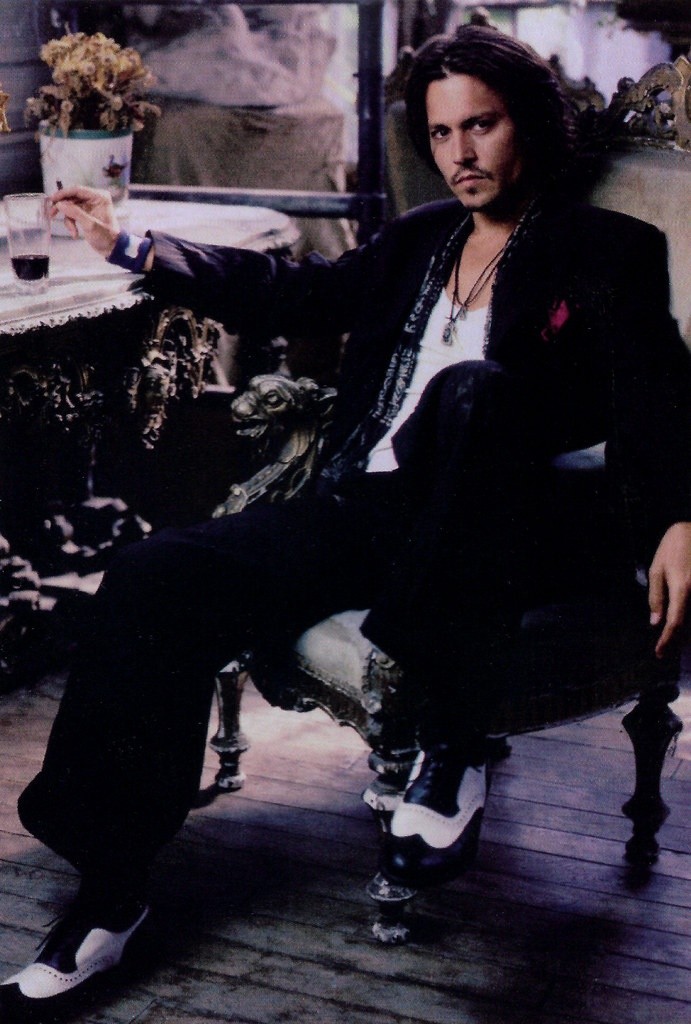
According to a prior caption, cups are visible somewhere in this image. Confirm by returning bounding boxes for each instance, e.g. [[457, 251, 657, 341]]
[[3, 191, 54, 295]]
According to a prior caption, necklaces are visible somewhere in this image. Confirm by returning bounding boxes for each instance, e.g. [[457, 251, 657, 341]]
[[441, 246, 506, 344]]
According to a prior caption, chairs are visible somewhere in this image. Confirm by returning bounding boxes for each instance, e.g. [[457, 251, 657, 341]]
[[124, 6, 691, 946]]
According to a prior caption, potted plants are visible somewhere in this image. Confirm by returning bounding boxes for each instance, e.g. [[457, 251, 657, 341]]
[[17, 30, 163, 238]]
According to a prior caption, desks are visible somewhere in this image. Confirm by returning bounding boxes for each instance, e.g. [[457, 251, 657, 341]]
[[0, 195, 301, 701]]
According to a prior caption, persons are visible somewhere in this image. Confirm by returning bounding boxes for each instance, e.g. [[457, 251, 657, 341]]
[[0, 23, 690, 1024]]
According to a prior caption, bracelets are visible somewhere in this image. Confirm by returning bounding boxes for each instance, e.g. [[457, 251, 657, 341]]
[[108, 232, 150, 272]]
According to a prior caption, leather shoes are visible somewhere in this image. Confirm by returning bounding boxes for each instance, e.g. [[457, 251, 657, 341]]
[[380, 748, 488, 889], [0, 899, 157, 1024]]
